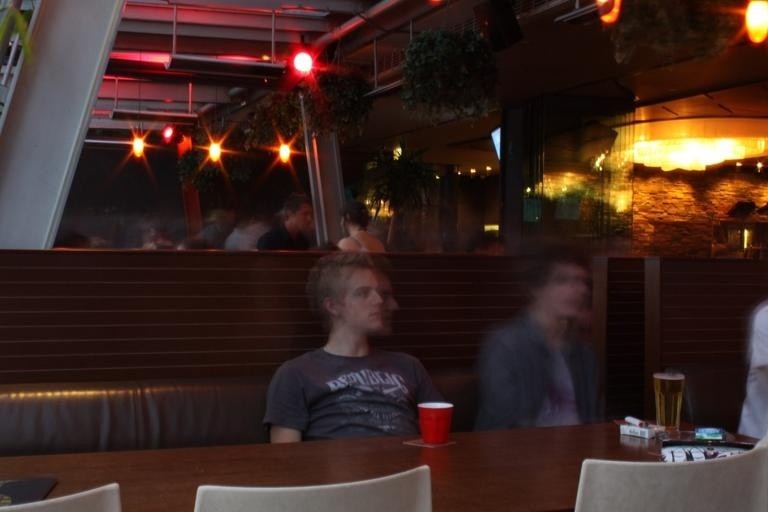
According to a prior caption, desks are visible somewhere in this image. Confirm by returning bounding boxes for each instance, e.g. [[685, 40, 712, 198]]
[[1, 411, 762, 512]]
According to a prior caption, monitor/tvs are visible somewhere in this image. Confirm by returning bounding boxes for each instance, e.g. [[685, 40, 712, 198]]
[[489, 125, 501, 160]]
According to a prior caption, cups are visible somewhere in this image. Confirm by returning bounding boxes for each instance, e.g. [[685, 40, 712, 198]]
[[417, 401, 454, 445], [652, 370, 685, 431]]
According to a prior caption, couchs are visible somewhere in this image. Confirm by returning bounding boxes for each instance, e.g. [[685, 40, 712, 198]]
[[0, 372, 480, 457], [652, 364, 747, 434]]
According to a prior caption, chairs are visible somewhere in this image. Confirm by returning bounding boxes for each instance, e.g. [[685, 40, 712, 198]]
[[193, 465, 432, 512], [573, 432, 768, 512], [1, 482, 123, 512]]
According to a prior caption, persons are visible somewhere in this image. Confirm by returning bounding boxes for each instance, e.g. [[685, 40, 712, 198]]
[[469, 249, 603, 432], [138, 218, 174, 252], [335, 201, 385, 252], [261, 248, 443, 445], [736, 299, 768, 441], [256, 191, 313, 251], [188, 206, 234, 245]]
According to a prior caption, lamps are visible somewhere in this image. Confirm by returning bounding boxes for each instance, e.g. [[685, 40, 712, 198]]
[[362, 19, 415, 100], [109, 78, 199, 124], [554, 0, 600, 23], [163, 2, 287, 79]]
